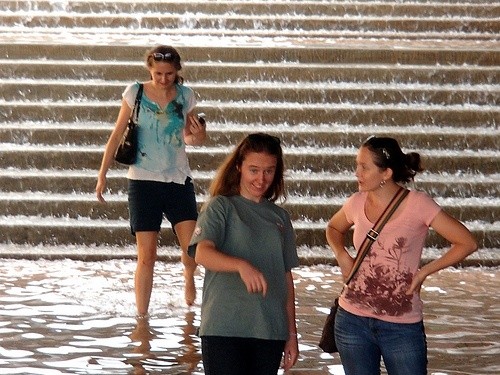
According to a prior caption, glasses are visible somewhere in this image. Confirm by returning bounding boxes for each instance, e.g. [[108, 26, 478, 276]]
[[238, 134, 281, 156], [367, 134, 393, 164], [150, 52, 180, 60]]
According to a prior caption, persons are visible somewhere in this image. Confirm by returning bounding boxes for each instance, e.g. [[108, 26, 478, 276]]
[[97, 46, 206, 337], [325, 137, 479, 375], [187, 132, 300, 374]]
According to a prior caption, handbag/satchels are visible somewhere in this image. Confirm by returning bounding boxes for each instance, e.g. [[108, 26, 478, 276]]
[[114, 83, 145, 165], [318, 299, 339, 353]]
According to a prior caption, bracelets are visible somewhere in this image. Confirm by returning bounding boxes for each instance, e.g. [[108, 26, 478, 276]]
[[289, 333, 297, 336]]
